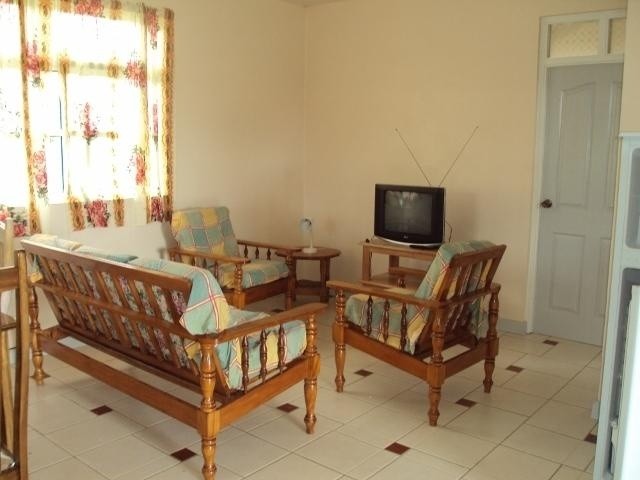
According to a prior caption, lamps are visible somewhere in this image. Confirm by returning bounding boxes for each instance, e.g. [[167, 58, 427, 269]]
[[299, 218, 318, 254]]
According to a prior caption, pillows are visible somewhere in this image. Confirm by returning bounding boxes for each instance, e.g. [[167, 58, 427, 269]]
[[28, 234, 243, 392], [404, 240, 497, 355], [171, 207, 240, 269]]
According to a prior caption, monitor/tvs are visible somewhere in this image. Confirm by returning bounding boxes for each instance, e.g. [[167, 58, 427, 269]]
[[374, 183, 446, 249]]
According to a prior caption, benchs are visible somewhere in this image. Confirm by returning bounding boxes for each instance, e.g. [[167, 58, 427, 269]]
[[20, 239, 329, 480]]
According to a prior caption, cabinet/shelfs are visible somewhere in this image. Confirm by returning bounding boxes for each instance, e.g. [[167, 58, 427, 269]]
[[362, 240, 441, 293]]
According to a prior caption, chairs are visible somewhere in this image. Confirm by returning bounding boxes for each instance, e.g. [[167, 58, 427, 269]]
[[167, 207, 295, 312], [325, 245, 507, 427]]
